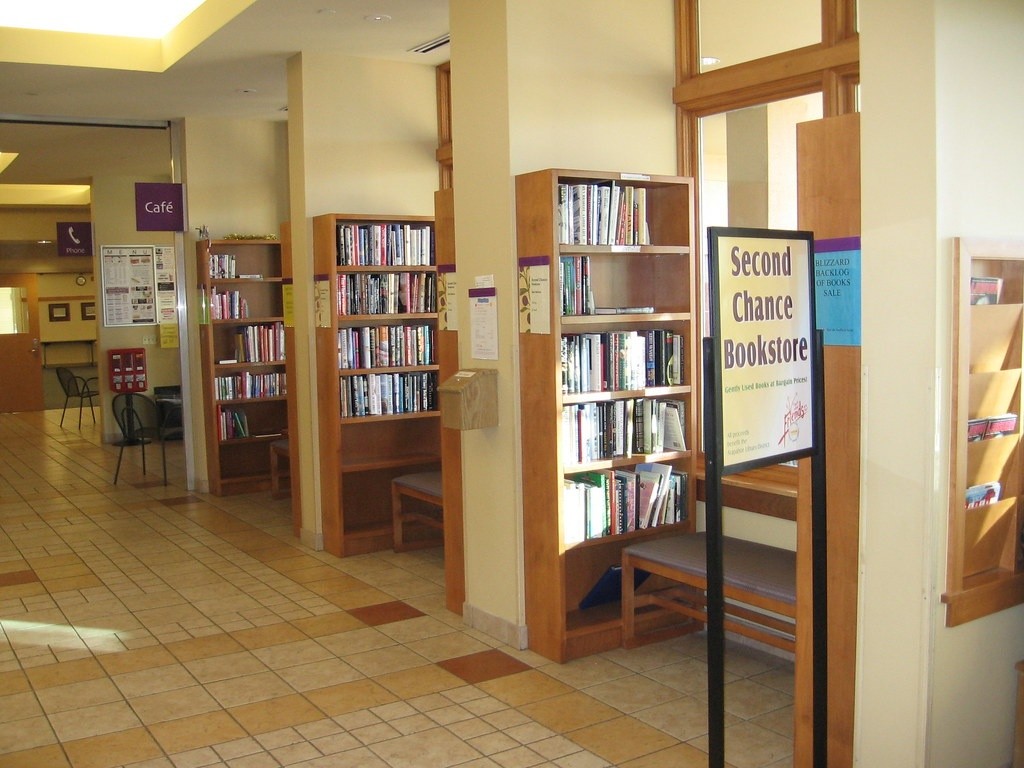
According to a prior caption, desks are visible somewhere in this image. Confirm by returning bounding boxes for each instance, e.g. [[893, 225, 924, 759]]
[[697, 452, 798, 521]]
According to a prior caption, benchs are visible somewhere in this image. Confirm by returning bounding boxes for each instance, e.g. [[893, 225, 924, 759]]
[[270, 439, 289, 495], [392, 471, 443, 552], [621, 533, 797, 654]]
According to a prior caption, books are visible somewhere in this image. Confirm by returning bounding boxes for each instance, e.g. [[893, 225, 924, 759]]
[[565, 465, 692, 545], [966, 480, 1000, 508], [340, 271, 436, 315], [337, 322, 433, 369], [210, 289, 247, 319], [208, 252, 260, 278], [560, 253, 658, 315], [970, 274, 1001, 307], [214, 370, 285, 399], [338, 374, 436, 417], [564, 399, 688, 464], [560, 329, 683, 394], [558, 183, 652, 247], [220, 407, 283, 441], [968, 411, 1019, 440], [336, 223, 436, 267], [215, 323, 287, 364]]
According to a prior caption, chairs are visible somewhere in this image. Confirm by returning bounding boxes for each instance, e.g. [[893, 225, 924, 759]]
[[111, 392, 183, 486], [56, 367, 100, 430]]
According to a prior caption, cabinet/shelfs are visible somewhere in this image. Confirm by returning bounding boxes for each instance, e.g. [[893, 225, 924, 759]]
[[312, 213, 443, 556], [942, 237, 1024, 628], [195, 239, 289, 497], [514, 168, 705, 664]]
